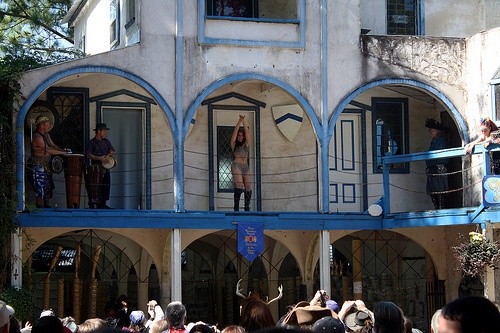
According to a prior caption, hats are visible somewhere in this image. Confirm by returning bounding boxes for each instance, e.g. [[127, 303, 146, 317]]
[[0, 301, 15, 327], [93, 123, 110, 130], [344, 310, 371, 332], [425, 119, 447, 131], [283, 301, 338, 325], [326, 300, 338, 312]]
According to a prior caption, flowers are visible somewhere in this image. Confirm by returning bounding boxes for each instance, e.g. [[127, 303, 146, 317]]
[[448, 233, 500, 283]]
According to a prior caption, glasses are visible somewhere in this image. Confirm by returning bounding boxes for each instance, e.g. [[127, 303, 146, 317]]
[[8, 315, 13, 320]]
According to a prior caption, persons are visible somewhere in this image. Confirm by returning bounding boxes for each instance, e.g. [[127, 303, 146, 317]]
[[84, 123, 117, 209], [0, 288, 443, 333], [28, 116, 72, 209], [463, 119, 500, 175], [230, 114, 252, 212], [425, 118, 450, 210], [438, 296, 500, 333]]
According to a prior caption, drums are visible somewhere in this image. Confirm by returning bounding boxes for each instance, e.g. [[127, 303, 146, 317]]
[[26, 155, 63, 174], [102, 155, 116, 169], [63, 154, 85, 209]]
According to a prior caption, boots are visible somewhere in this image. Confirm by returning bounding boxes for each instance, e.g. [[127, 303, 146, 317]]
[[43, 194, 52, 208], [234, 188, 242, 211], [35, 197, 43, 207], [244, 190, 252, 211]]
